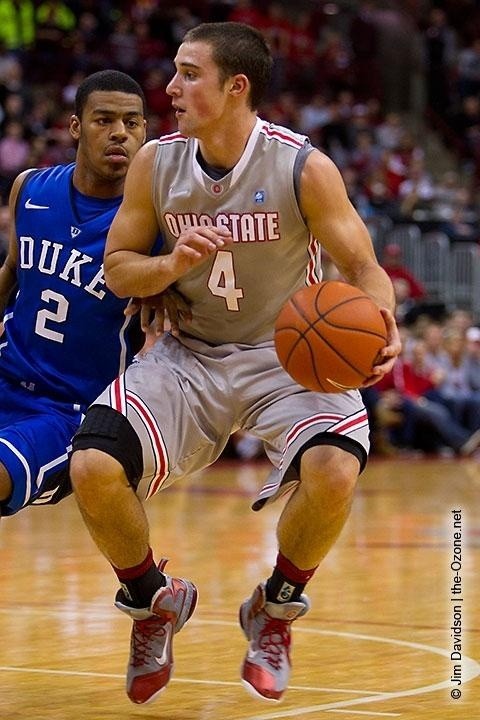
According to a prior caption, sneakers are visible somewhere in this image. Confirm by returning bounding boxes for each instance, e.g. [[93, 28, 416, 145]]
[[236, 578, 310, 705], [113, 558, 198, 706]]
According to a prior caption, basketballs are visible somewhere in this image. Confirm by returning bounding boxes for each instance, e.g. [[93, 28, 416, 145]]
[[274, 280, 387, 393]]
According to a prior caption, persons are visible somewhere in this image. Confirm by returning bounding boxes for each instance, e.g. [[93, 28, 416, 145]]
[[66, 20, 406, 708], [1, 70, 192, 519], [0, 0, 480, 463]]
